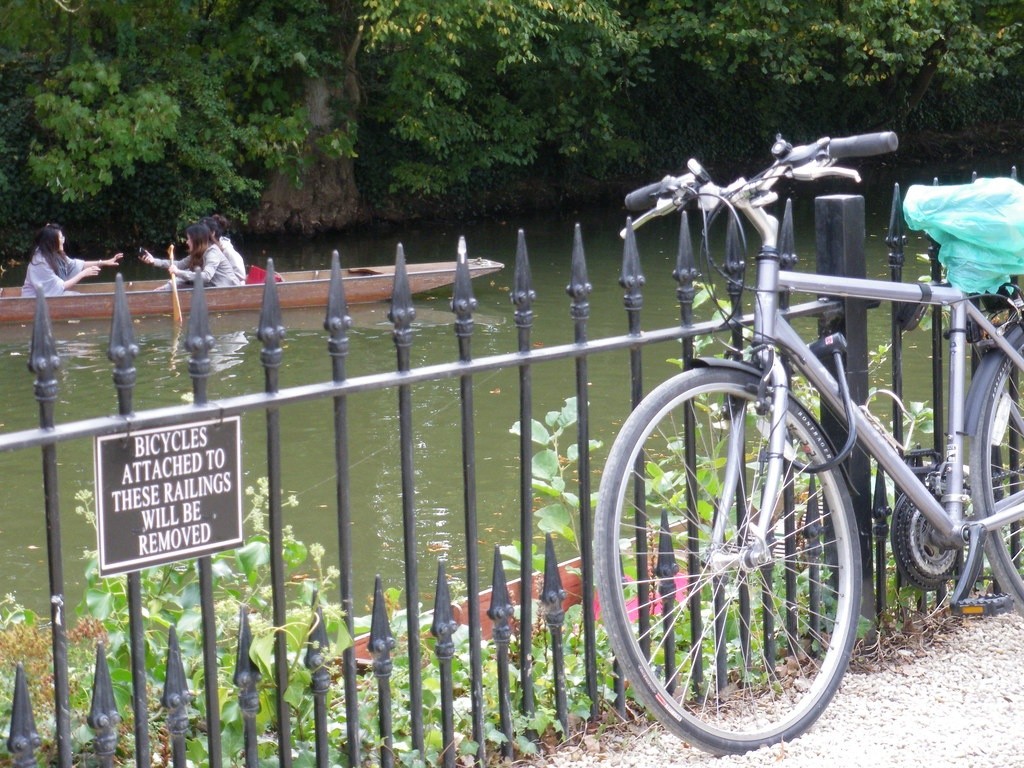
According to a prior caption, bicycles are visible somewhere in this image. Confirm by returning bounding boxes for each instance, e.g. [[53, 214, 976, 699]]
[[593, 131, 1024, 758]]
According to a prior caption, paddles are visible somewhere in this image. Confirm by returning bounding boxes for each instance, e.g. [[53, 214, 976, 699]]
[[166, 244, 183, 322]]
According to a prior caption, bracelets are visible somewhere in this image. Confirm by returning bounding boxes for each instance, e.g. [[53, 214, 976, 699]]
[[99, 259, 101, 265]]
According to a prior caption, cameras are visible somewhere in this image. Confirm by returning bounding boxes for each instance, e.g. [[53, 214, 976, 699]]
[[136, 247, 147, 257]]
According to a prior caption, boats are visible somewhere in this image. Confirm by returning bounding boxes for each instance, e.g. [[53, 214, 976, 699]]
[[0, 257, 506, 322]]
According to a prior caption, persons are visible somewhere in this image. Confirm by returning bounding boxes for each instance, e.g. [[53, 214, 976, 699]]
[[21, 223, 123, 297], [154, 214, 247, 291], [138, 223, 241, 288]]
